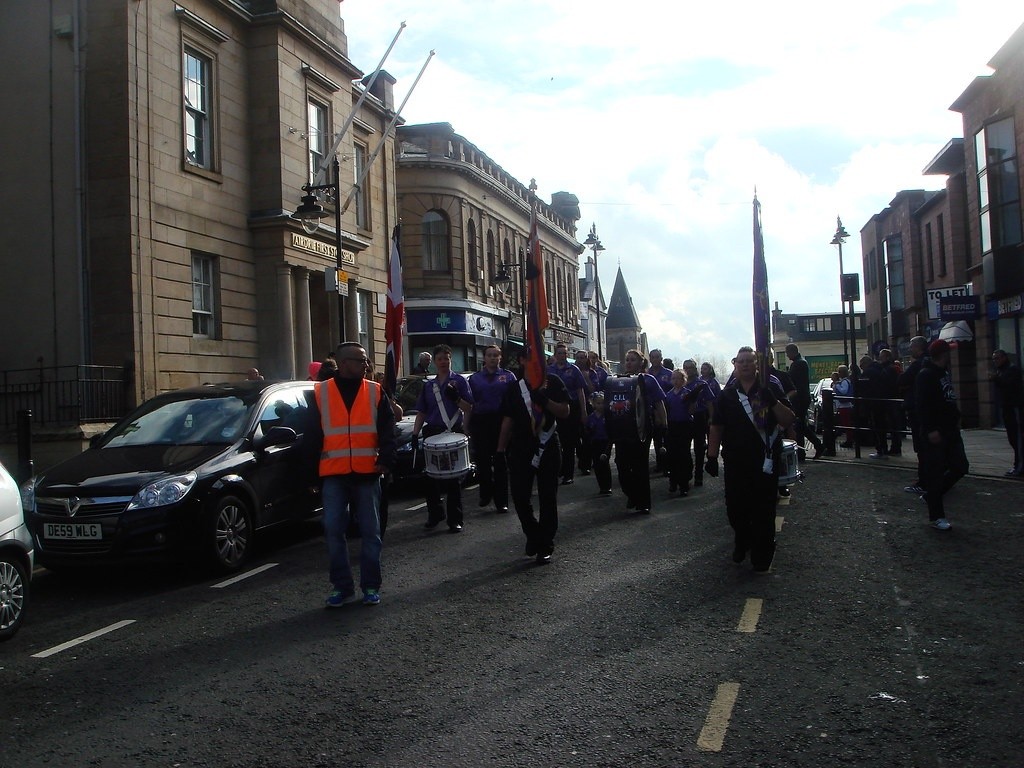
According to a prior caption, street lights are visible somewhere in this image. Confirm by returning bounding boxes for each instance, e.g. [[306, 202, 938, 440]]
[[291, 153, 348, 343], [583, 224, 606, 360], [829, 216, 852, 370], [491, 245, 528, 358]]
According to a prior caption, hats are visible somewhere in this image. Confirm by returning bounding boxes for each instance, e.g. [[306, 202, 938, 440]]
[[927, 339, 959, 356]]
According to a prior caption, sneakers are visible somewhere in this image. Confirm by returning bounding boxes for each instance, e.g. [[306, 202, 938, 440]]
[[916, 495, 928, 506], [363, 583, 380, 605], [928, 517, 953, 530], [325, 588, 356, 606], [904, 482, 926, 493]]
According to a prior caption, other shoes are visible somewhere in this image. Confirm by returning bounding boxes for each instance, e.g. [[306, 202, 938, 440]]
[[558, 452, 702, 496], [798, 439, 905, 462], [479, 489, 493, 507], [535, 548, 551, 565], [642, 508, 649, 514], [1004, 467, 1024, 479], [778, 485, 790, 496], [626, 497, 636, 508], [423, 513, 446, 529], [497, 505, 509, 513], [525, 533, 540, 555], [448, 521, 464, 532], [751, 550, 777, 574], [732, 544, 750, 562]]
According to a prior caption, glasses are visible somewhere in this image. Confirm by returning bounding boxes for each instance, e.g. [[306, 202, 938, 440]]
[[340, 357, 370, 367], [734, 359, 756, 366]]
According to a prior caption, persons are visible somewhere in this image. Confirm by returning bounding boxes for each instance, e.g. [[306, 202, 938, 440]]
[[411, 344, 474, 532], [706, 342, 824, 571], [829, 336, 969, 500], [495, 349, 572, 562], [461, 343, 723, 515], [912, 340, 965, 531], [247, 367, 264, 380], [989, 349, 1024, 476], [302, 342, 403, 606], [410, 352, 432, 375]]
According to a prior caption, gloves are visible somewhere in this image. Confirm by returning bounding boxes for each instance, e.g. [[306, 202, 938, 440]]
[[770, 430, 784, 454], [705, 454, 719, 479]]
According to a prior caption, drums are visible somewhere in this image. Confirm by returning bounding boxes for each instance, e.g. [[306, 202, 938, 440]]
[[777, 439, 802, 485], [603, 372, 649, 445], [422, 432, 471, 479]]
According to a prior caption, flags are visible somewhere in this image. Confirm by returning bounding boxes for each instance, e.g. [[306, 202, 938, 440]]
[[525, 210, 551, 390], [382, 225, 405, 398], [753, 198, 772, 420]]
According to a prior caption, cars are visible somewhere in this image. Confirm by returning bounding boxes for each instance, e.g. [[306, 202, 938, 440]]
[[805, 377, 840, 436], [18, 380, 400, 579], [392, 369, 476, 487], [0, 459, 36, 644]]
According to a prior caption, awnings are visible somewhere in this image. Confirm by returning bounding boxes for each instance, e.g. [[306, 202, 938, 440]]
[[939, 320, 973, 343]]
